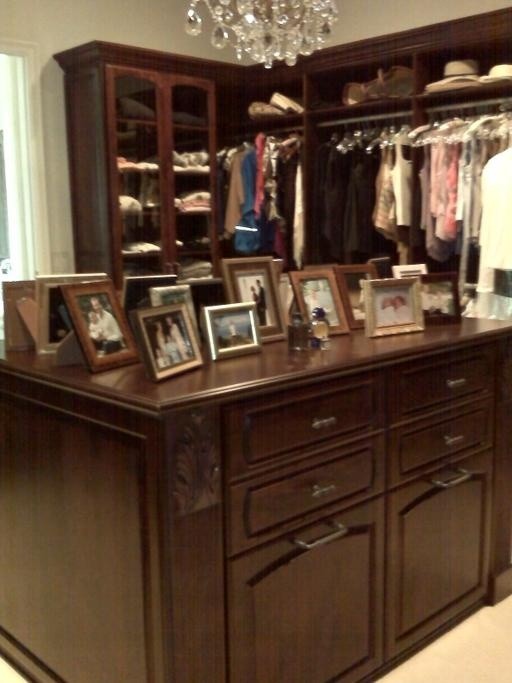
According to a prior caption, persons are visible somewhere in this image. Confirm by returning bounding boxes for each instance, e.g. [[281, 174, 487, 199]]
[[358, 280, 368, 314], [88, 295, 124, 356], [420, 283, 452, 316], [228, 319, 241, 343], [379, 296, 412, 325], [307, 287, 334, 322], [149, 315, 193, 369], [250, 280, 268, 327]]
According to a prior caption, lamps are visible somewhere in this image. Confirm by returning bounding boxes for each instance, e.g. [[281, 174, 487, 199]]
[[183, 2, 340, 70]]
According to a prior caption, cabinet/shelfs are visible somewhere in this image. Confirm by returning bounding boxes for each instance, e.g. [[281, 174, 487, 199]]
[[0, 318, 511, 683], [52, 5, 512, 287]]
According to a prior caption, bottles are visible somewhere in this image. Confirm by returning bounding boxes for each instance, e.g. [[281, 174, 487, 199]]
[[309, 307, 332, 348], [288, 311, 310, 353]]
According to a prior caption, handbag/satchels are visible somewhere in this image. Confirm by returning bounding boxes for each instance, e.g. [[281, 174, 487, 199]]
[[343, 66, 414, 106]]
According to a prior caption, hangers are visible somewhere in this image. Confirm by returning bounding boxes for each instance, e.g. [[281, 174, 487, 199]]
[[226, 124, 303, 157], [320, 100, 512, 156]]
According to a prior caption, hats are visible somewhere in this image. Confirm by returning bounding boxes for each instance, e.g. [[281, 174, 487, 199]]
[[478, 64, 512, 83], [425, 60, 481, 90]]
